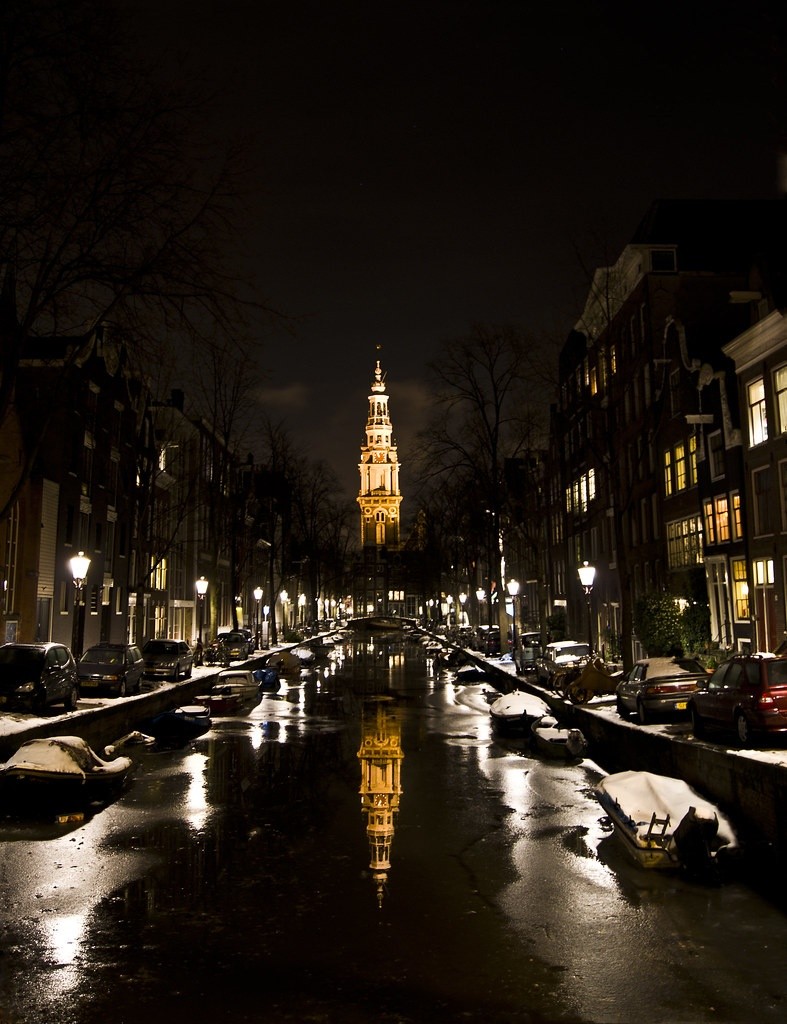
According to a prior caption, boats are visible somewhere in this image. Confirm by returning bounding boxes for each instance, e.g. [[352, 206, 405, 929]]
[[163, 629, 353, 729], [531, 715, 589, 764], [595, 770, 739, 875], [489, 688, 552, 736], [0, 735, 132, 825], [399, 620, 486, 681]]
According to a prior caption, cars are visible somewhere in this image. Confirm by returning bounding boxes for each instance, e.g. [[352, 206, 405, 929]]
[[142, 639, 193, 682], [534, 640, 591, 689], [614, 657, 713, 725], [217, 633, 249, 661], [469, 624, 499, 652], [513, 631, 540, 673], [230, 629, 256, 655]]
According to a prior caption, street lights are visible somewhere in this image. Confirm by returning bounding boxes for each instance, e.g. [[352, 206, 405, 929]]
[[476, 587, 485, 626], [577, 561, 597, 656], [254, 587, 264, 649], [507, 578, 520, 661], [195, 576, 209, 666], [280, 590, 288, 635], [69, 551, 92, 658], [446, 593, 467, 626], [299, 594, 306, 625]]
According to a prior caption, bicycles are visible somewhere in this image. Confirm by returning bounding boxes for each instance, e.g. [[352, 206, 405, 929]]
[[548, 655, 624, 706]]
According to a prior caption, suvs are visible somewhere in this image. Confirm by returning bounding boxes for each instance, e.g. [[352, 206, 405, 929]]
[[0, 642, 79, 715], [77, 642, 145, 697], [686, 652, 787, 748]]
